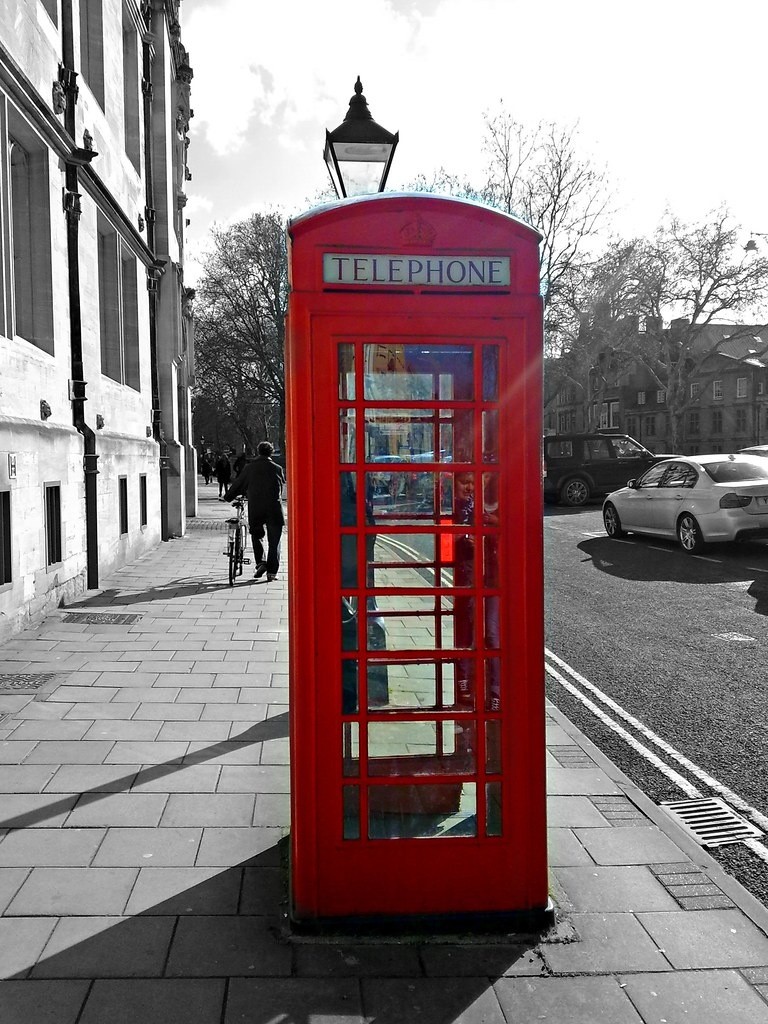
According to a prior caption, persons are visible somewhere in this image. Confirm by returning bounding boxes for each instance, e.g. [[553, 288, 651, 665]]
[[454, 462, 500, 720], [333, 419, 389, 715], [233, 452, 249, 499], [202, 459, 214, 485], [214, 454, 232, 497], [219, 442, 286, 581]]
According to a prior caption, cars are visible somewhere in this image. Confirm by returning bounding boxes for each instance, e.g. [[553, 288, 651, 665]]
[[602, 445, 768, 555], [370, 450, 453, 505]]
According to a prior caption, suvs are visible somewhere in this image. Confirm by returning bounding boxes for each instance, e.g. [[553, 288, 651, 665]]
[[544, 433, 685, 507]]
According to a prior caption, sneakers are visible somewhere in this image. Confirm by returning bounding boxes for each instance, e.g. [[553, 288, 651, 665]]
[[489, 695, 500, 713], [454, 677, 471, 698]]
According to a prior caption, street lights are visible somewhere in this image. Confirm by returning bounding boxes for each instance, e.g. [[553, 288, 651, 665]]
[[322, 75, 401, 709], [200, 434, 205, 459]]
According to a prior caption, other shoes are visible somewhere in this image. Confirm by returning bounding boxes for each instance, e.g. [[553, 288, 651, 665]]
[[254, 563, 268, 578], [266, 576, 278, 581]]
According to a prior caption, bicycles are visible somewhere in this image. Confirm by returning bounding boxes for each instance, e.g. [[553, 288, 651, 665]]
[[223, 495, 252, 586]]
